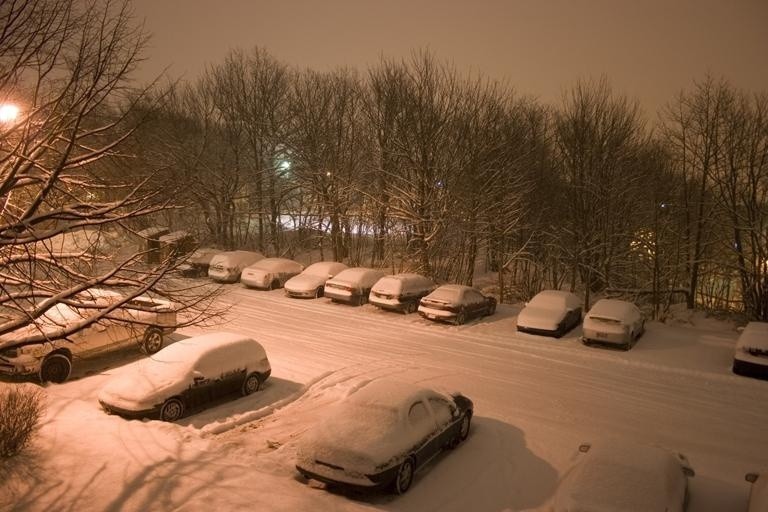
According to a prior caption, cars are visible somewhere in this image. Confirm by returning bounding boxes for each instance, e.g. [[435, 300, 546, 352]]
[[732, 321, 768, 374], [516, 289, 645, 351]]
